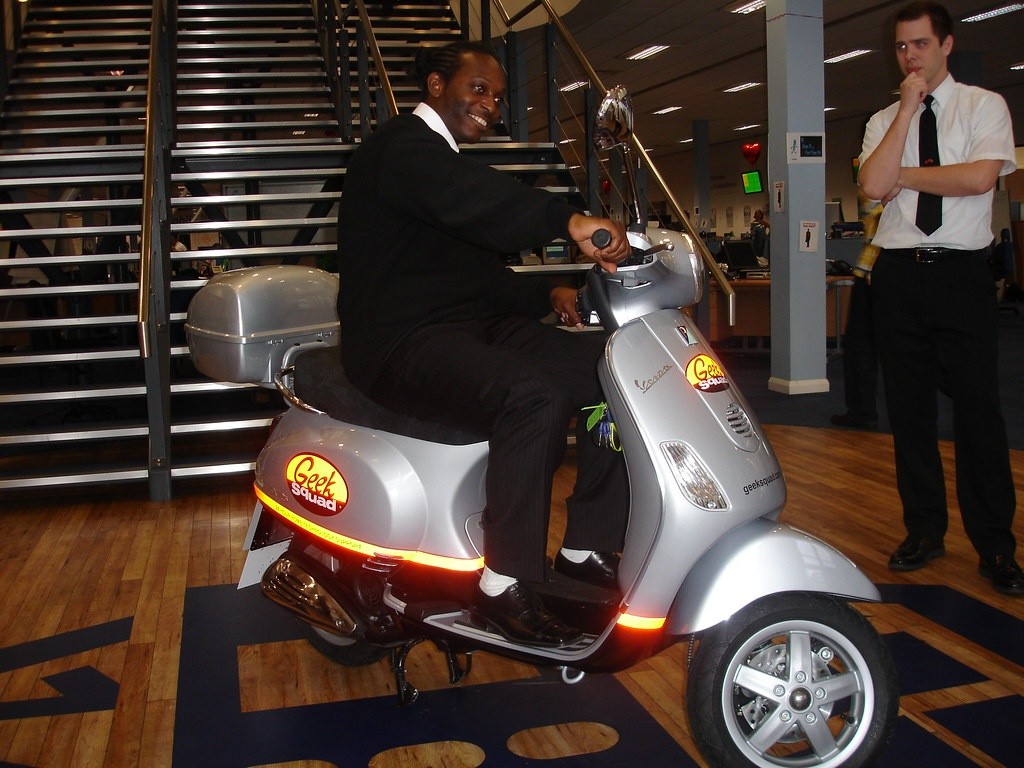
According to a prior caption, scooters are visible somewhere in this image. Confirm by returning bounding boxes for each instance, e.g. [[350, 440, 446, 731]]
[[186, 86, 900, 767]]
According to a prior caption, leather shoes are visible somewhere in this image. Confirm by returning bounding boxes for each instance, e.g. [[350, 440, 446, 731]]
[[886, 534, 944, 572], [978, 556, 1024, 596], [555, 548, 623, 592], [469, 580, 584, 646]]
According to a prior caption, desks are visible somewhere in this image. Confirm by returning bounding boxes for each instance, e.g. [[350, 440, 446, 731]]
[[687, 276, 870, 347]]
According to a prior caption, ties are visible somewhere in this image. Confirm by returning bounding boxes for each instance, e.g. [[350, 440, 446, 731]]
[[916, 95, 942, 237]]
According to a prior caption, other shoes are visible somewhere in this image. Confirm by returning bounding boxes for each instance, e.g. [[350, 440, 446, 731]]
[[830, 413, 879, 430]]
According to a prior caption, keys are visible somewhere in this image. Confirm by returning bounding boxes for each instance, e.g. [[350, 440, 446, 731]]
[[599, 413, 610, 448]]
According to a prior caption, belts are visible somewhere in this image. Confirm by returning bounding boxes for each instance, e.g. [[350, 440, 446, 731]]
[[882, 247, 980, 264]]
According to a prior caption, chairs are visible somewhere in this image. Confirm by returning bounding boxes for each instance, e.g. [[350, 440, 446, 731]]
[[721, 239, 759, 270]]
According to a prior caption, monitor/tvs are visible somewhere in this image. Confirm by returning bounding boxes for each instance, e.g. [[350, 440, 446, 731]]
[[741, 170, 763, 195], [724, 240, 760, 270]]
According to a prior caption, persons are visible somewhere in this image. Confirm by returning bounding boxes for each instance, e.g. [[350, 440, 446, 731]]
[[749, 209, 770, 239], [857, 0, 1024, 596], [171, 232, 191, 275], [831, 180, 888, 429], [336, 41, 633, 647]]
